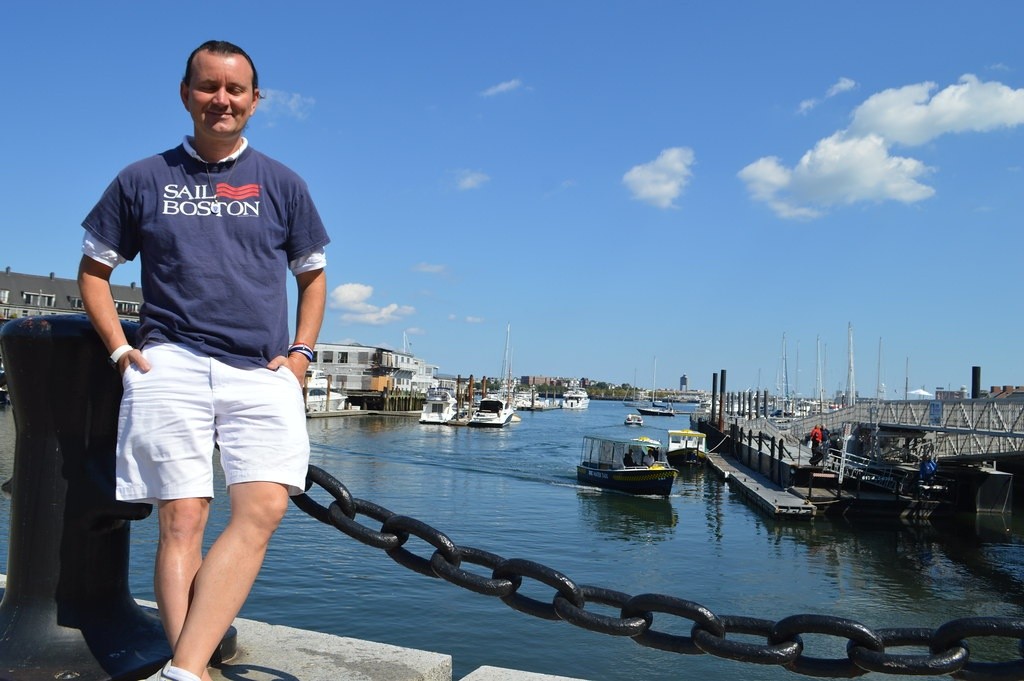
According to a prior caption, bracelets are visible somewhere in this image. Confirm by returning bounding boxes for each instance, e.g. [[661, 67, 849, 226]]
[[288, 343, 314, 362], [108, 344, 134, 371]]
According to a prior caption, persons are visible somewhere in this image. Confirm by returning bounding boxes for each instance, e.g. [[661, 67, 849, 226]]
[[810, 424, 831, 445], [652, 447, 659, 461], [642, 449, 654, 468], [77, 39, 330, 681], [623, 449, 639, 467]]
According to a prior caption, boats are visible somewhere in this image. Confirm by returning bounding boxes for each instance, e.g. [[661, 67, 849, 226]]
[[302, 365, 348, 411], [667, 429, 708, 469], [463, 382, 544, 410], [624, 415, 644, 427], [559, 379, 590, 409], [418, 384, 457, 425], [576, 433, 679, 499]]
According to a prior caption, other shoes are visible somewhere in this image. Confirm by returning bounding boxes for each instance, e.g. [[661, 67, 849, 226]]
[[138, 659, 178, 681]]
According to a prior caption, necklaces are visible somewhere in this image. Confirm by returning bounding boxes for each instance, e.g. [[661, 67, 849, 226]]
[[196, 136, 242, 215]]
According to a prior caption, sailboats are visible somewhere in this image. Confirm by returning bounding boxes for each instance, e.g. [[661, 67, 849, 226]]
[[469, 320, 514, 427], [621, 366, 649, 408], [699, 321, 855, 420], [636, 356, 675, 417], [654, 385, 662, 403]]
